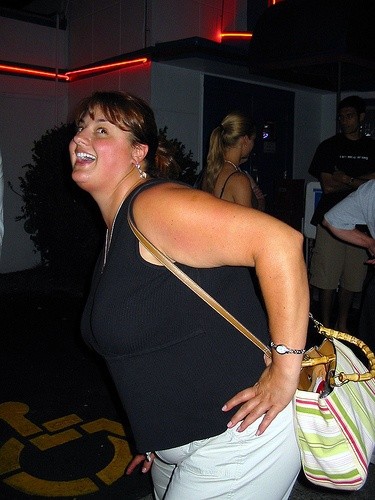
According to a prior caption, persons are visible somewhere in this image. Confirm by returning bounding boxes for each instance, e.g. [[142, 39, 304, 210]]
[[194, 112, 266, 212], [307, 95, 375, 347], [68, 91, 310, 500], [322, 178, 375, 264]]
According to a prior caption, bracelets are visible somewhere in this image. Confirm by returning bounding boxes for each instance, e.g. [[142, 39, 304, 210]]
[[348, 177, 354, 186]]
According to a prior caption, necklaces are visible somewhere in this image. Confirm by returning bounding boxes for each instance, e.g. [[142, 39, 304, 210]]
[[99, 196, 128, 274], [224, 159, 238, 172]]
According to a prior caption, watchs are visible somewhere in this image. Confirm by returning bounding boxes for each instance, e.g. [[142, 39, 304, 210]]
[[270, 342, 305, 355]]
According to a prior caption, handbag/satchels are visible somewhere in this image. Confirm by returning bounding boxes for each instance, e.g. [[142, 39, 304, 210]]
[[292, 310, 375, 490]]
